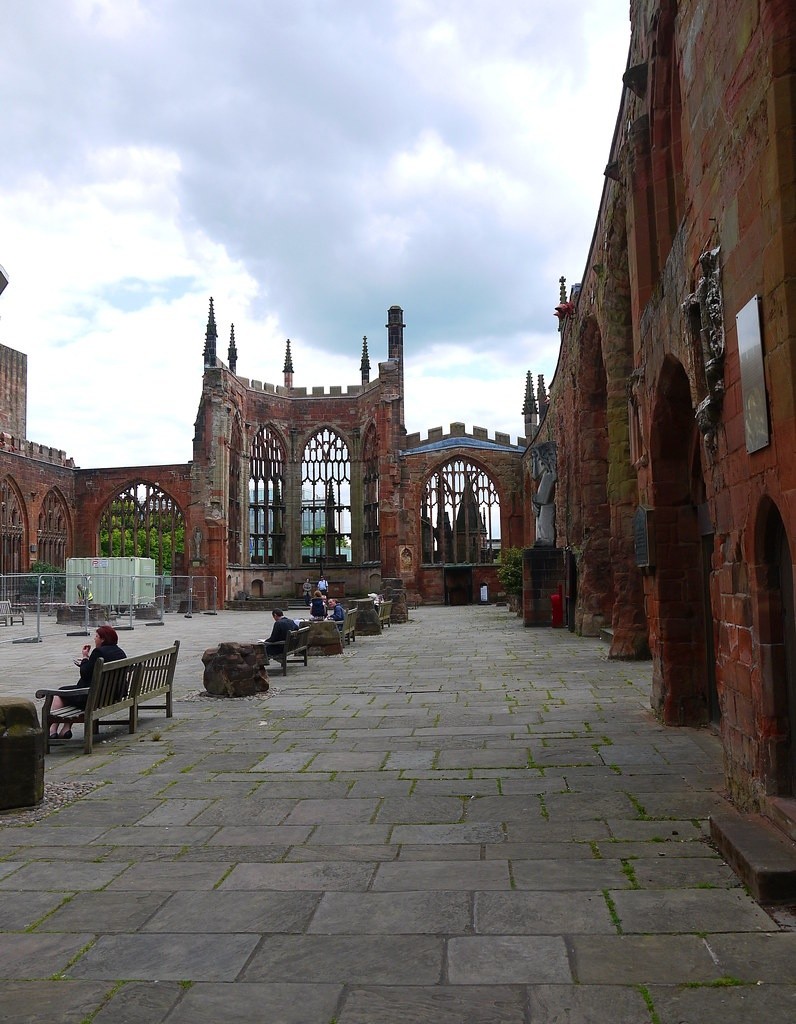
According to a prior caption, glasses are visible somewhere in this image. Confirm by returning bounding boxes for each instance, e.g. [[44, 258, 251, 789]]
[[320, 577, 323, 578]]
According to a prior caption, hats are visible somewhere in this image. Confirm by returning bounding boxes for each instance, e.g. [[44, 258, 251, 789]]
[[368, 593, 377, 598], [97, 626, 118, 645]]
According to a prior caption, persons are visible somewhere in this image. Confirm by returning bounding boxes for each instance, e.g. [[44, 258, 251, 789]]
[[310, 591, 327, 619], [303, 578, 312, 607], [317, 576, 328, 595], [327, 599, 346, 631], [368, 593, 384, 606], [50, 626, 127, 745], [263, 608, 298, 666], [530, 441, 558, 546], [77, 584, 93, 604]]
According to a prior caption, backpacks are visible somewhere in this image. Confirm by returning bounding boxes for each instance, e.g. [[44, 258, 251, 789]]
[[311, 598, 325, 616]]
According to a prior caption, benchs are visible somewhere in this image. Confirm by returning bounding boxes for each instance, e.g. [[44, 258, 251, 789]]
[[35, 640, 180, 757], [375, 598, 393, 629], [0, 598, 26, 626], [333, 605, 359, 649], [256, 624, 312, 676]]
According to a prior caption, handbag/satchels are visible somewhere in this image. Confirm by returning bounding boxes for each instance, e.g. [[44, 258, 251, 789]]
[[323, 589, 328, 595]]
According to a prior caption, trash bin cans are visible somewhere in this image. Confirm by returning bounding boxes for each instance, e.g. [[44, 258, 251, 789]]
[[548, 594, 563, 628]]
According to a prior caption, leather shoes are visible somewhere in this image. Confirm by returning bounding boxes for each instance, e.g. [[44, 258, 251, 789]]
[[59, 730, 72, 739], [48, 733, 58, 739]]
